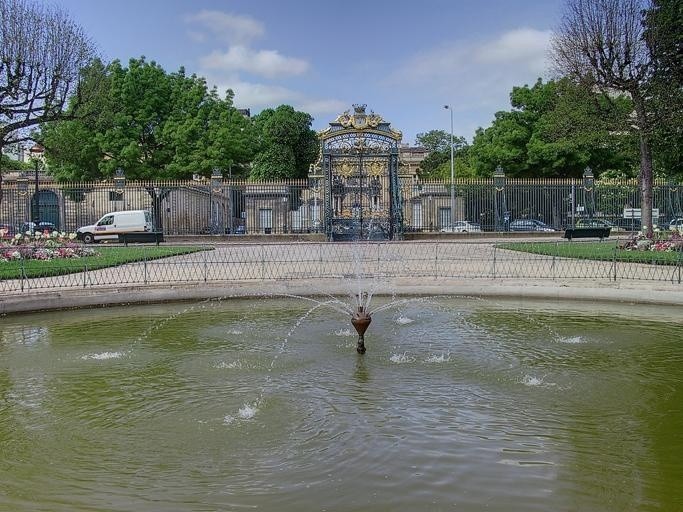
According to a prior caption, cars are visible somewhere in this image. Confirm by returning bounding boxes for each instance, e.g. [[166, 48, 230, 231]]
[[440, 220, 480, 232], [1, 221, 55, 237], [510, 218, 546, 230], [657, 218, 683, 231], [200, 223, 218, 235], [612, 218, 641, 230], [232, 225, 245, 234]]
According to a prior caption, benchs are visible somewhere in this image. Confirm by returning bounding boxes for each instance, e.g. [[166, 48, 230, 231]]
[[118, 231, 166, 247], [561, 228, 610, 241]]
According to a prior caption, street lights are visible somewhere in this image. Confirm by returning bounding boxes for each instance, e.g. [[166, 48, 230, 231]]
[[30, 143, 45, 241], [443, 105, 455, 231]]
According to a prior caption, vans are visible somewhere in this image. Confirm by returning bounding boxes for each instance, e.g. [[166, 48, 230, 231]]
[[575, 218, 617, 231], [75, 211, 154, 244]]
